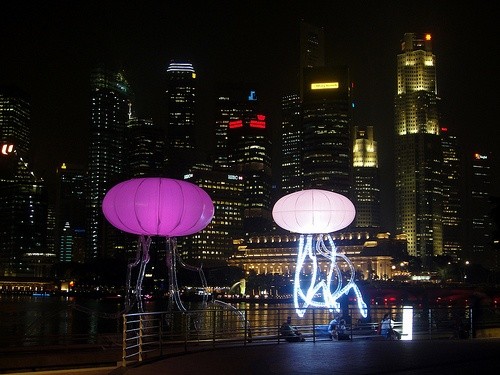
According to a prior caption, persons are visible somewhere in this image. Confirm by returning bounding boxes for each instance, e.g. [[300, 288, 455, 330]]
[[278, 310, 404, 342]]
